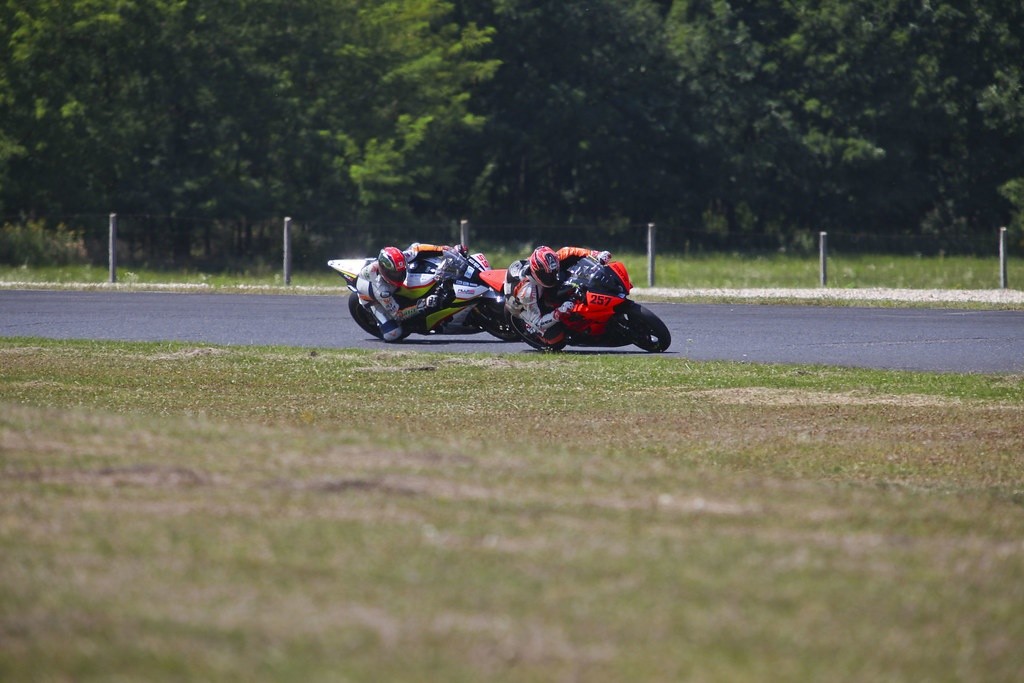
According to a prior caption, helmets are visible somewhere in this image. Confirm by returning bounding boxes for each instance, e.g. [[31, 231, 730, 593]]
[[377, 247, 407, 287], [529, 246, 560, 287]]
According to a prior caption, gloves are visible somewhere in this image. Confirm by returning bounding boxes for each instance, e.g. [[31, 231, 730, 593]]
[[554, 301, 575, 320], [442, 243, 467, 255], [417, 294, 440, 314], [590, 250, 611, 265]]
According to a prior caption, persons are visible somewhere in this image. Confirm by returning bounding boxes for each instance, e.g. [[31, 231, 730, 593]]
[[355, 242, 469, 341], [504, 246, 611, 351]]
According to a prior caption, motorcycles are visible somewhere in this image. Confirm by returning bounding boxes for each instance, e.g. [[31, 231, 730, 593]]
[[480, 252, 671, 355], [326, 248, 526, 343]]
